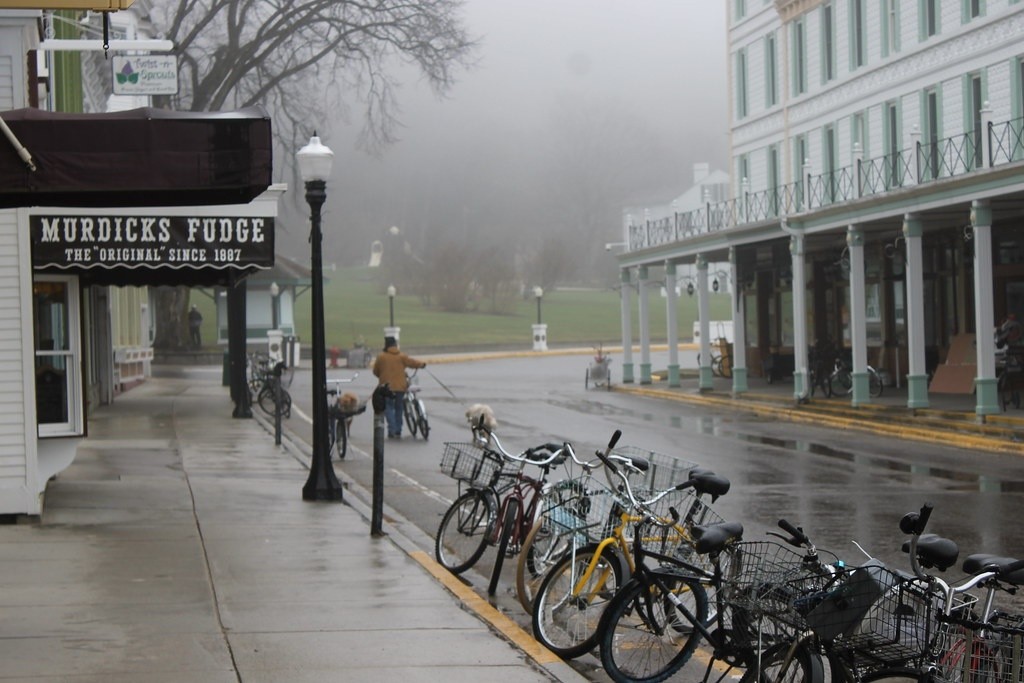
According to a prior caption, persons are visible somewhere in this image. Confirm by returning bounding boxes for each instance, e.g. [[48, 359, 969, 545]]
[[189, 304, 203, 347], [997, 313, 1024, 372], [373, 336, 426, 438]]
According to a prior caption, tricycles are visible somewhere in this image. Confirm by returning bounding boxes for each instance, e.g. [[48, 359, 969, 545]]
[[583, 342, 613, 393]]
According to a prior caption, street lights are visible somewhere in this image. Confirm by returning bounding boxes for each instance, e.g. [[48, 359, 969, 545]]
[[295, 129, 344, 503], [383, 284, 401, 353], [530, 286, 549, 351], [269, 281, 284, 374]]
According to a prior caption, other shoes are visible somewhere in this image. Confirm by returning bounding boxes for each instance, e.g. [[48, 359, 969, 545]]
[[389, 432, 394, 437], [396, 431, 401, 435]]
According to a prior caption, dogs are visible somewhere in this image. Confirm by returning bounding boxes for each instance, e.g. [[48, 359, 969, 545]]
[[465, 403, 497, 445], [337, 392, 358, 438]]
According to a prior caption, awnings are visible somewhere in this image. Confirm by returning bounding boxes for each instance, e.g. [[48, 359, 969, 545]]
[[0, 106, 272, 213], [29, 214, 274, 289]]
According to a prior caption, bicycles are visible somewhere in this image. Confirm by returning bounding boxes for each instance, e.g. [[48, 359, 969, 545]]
[[404, 367, 431, 440], [244, 348, 292, 420], [698, 333, 883, 400], [435, 403, 1023, 683], [324, 373, 360, 459]]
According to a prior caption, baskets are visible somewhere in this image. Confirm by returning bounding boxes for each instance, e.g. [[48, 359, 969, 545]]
[[785, 564, 980, 683], [258, 359, 279, 382], [931, 614, 1024, 683], [611, 444, 699, 503], [721, 542, 831, 650], [631, 488, 727, 576], [540, 477, 630, 546], [439, 441, 520, 496]]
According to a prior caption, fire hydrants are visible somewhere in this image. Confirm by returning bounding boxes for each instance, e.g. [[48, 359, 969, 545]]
[[327, 345, 342, 368]]
